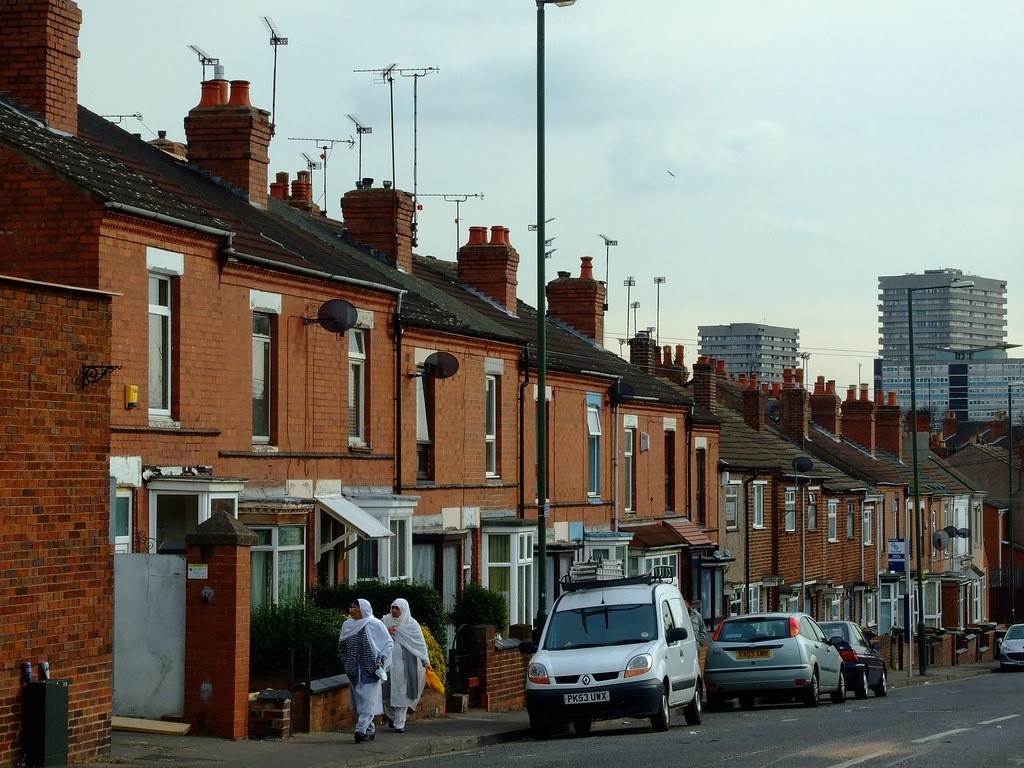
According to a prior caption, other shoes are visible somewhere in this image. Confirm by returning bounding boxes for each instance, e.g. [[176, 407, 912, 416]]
[[397, 728, 405, 733], [354, 731, 363, 743], [388, 719, 394, 727], [363, 731, 376, 740]]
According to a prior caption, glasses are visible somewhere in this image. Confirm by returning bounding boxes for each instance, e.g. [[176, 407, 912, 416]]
[[349, 608, 360, 612]]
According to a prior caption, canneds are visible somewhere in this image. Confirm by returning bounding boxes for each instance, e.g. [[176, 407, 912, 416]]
[[23, 661, 32, 683], [40, 661, 50, 680]]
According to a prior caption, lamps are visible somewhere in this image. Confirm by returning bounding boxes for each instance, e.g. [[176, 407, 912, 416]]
[[406, 351, 459, 379], [603, 383, 635, 407], [792, 456, 813, 488], [931, 525, 971, 551], [304, 298, 357, 339]]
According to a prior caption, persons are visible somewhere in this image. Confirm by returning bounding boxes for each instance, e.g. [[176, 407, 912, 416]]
[[337, 599, 394, 744], [381, 598, 434, 733]]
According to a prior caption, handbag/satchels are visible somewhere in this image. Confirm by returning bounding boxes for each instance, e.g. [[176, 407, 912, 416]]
[[427, 669, 445, 693], [375, 666, 387, 680]]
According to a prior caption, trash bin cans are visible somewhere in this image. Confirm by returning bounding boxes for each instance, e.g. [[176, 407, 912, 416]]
[[448, 648, 481, 707], [21, 678, 68, 768]]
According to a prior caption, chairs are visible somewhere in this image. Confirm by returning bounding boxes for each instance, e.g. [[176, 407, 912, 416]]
[[742, 626, 756, 639]]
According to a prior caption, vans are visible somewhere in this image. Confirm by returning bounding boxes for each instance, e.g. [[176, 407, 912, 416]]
[[524, 583, 703, 732]]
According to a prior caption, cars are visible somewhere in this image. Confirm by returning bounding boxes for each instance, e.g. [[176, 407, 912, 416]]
[[703, 612, 846, 708], [997, 623, 1024, 669], [815, 621, 888, 697]]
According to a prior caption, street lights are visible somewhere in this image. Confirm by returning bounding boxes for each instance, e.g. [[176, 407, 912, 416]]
[[907, 280, 976, 671], [536, 0, 576, 645], [1007, 381, 1024, 623]]
